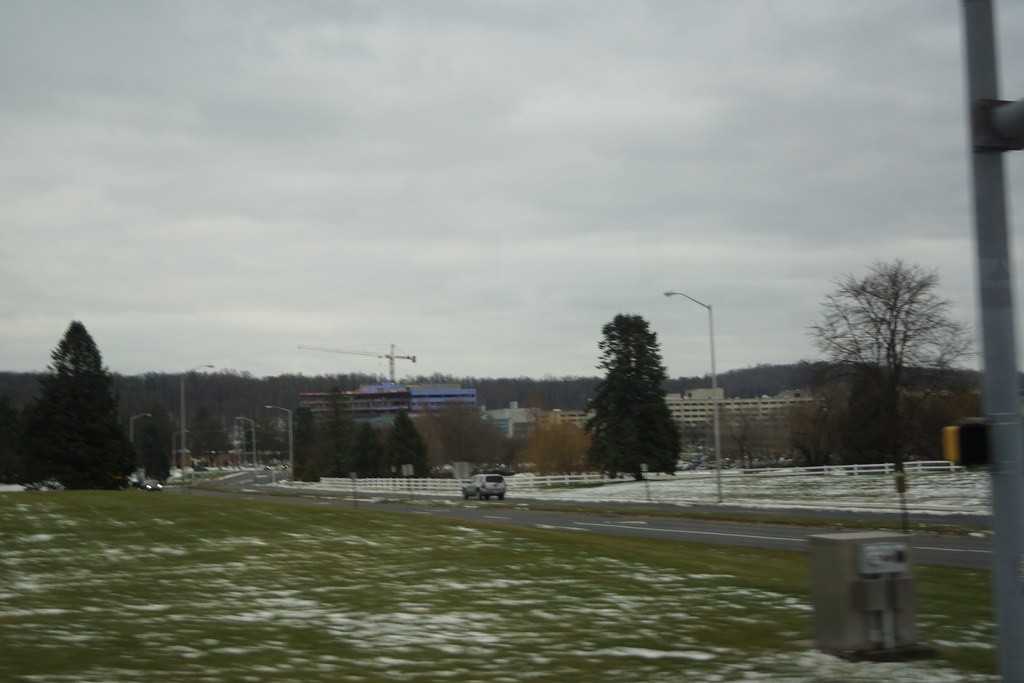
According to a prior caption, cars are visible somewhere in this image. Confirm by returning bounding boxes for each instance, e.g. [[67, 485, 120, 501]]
[[139, 479, 162, 492]]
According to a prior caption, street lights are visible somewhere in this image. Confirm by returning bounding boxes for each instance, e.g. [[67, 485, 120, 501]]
[[130, 413, 152, 442], [181, 364, 216, 493], [665, 291, 723, 506], [235, 417, 257, 468], [265, 405, 294, 484]]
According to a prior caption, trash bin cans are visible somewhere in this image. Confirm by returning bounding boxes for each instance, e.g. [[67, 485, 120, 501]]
[[810, 530, 915, 661]]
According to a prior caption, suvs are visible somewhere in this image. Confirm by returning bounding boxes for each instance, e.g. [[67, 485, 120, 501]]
[[461, 473, 507, 500]]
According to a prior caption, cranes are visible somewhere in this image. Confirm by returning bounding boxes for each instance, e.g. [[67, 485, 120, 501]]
[[297, 344, 416, 383]]
